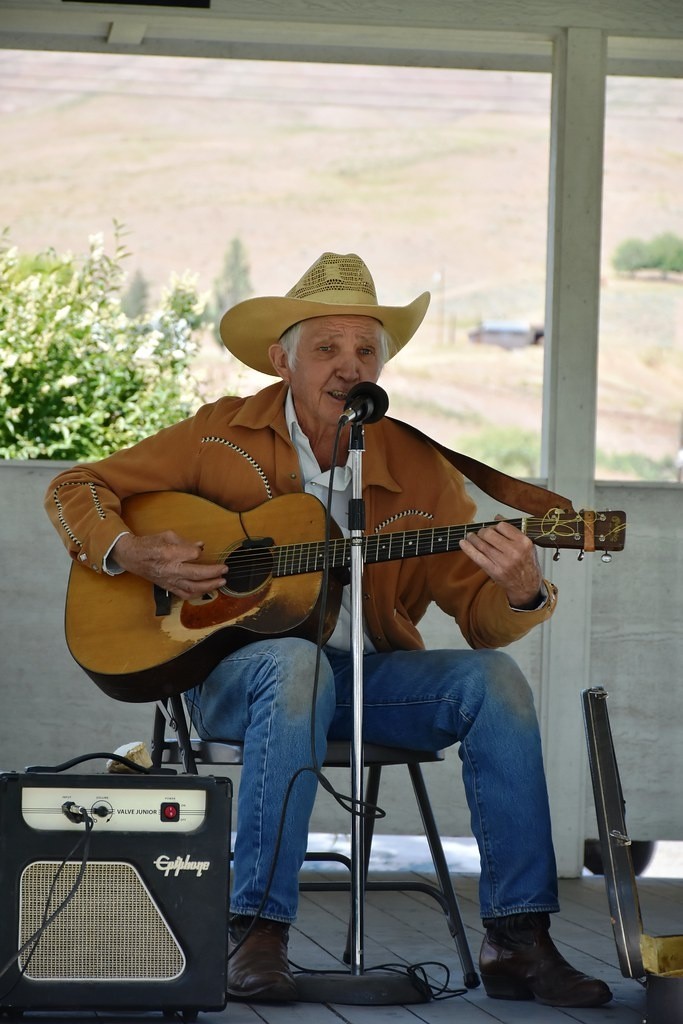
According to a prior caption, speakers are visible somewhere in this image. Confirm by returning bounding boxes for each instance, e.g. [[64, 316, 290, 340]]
[[0, 770, 233, 1012]]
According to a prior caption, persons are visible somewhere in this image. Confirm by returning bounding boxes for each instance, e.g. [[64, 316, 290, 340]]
[[43, 250, 613, 1010]]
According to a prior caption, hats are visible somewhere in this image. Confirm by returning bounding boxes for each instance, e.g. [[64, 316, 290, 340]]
[[220, 252, 431, 378]]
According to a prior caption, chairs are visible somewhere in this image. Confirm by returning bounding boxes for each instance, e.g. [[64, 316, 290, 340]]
[[149, 674, 480, 987]]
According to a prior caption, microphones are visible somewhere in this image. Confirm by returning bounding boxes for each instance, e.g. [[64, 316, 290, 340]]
[[340, 382, 389, 427]]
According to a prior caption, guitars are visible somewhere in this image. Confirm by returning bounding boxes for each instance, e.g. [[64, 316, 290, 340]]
[[63, 490, 627, 704]]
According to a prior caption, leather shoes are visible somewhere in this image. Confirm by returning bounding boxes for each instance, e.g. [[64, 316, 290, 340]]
[[479, 931, 612, 1006], [220, 913, 297, 1006]]
